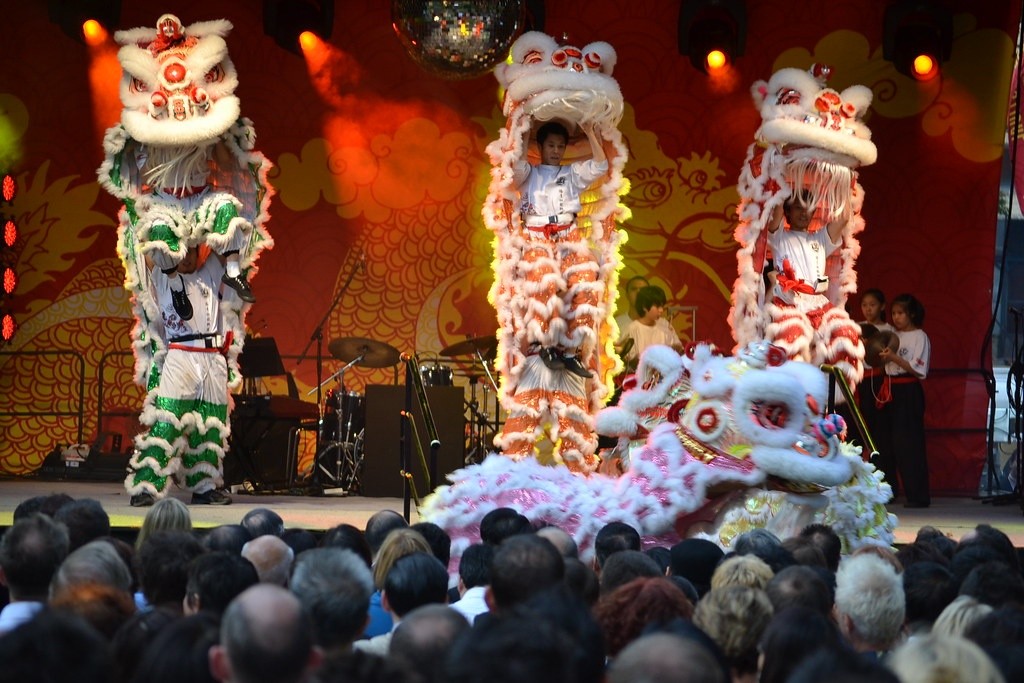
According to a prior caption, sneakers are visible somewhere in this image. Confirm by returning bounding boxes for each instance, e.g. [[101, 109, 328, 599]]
[[191, 488, 232, 505], [562, 354, 593, 378], [130, 492, 154, 506], [170, 273, 193, 320], [222, 268, 256, 303], [539, 346, 564, 371]]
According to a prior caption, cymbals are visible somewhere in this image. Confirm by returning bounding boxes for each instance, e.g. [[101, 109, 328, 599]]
[[453, 365, 494, 380], [327, 336, 400, 368], [439, 334, 498, 356], [859, 324, 900, 368], [614, 333, 634, 358]]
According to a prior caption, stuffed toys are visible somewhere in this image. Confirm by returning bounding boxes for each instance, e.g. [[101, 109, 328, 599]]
[[97, 15, 274, 491], [728, 61, 878, 393], [482, 27, 630, 477], [417, 339, 900, 590]]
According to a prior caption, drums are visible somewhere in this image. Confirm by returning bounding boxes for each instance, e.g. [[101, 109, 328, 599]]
[[321, 388, 363, 443], [419, 364, 454, 386], [315, 441, 360, 495]]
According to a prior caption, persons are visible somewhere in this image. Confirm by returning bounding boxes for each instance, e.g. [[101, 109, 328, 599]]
[[854, 288, 933, 510], [512, 119, 609, 378], [0, 491, 1024, 683], [124, 140, 258, 506], [763, 187, 851, 314], [614, 275, 685, 362]]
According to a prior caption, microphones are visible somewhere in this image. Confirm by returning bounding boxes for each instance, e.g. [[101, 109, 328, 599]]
[[359, 248, 367, 275]]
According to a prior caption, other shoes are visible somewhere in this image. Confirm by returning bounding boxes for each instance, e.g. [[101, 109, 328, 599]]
[[903, 499, 931, 508]]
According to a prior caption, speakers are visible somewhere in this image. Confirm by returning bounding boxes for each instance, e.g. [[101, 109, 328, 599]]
[[361, 382, 466, 498], [223, 414, 298, 489]]
